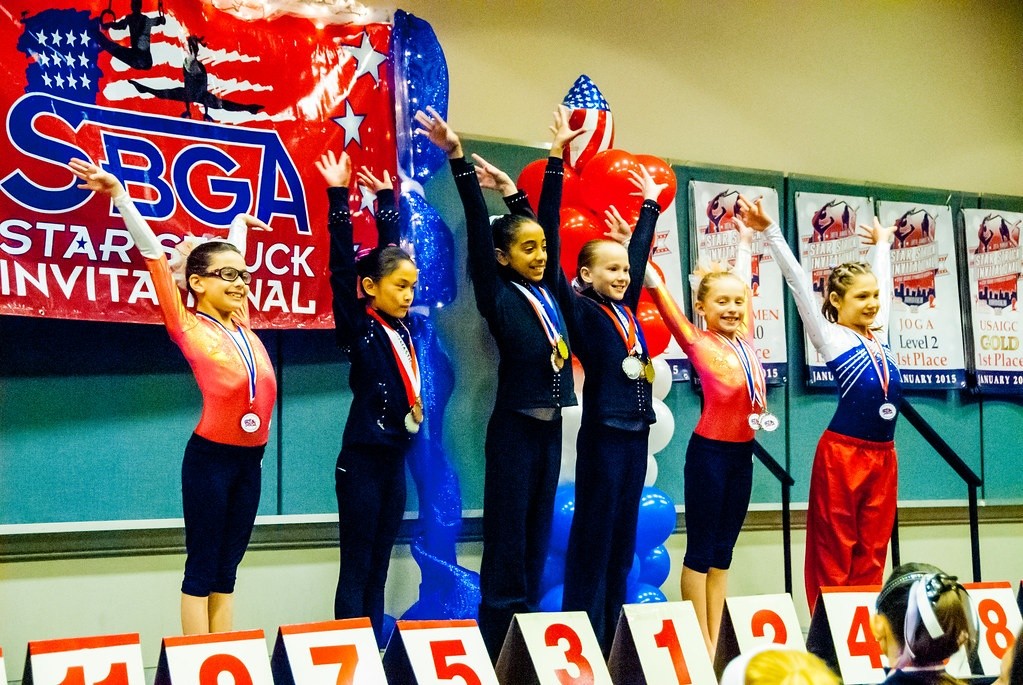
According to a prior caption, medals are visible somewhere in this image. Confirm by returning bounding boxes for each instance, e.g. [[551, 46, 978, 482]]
[[241, 413, 260, 432], [878, 404, 897, 420], [550, 339, 569, 371], [748, 412, 777, 431], [404, 404, 424, 434], [622, 357, 654, 382]]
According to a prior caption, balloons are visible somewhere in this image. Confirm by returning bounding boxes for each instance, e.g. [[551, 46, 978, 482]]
[[516, 76, 672, 364], [542, 360, 678, 610]]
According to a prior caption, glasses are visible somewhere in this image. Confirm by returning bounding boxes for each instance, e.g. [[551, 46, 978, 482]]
[[198, 267, 251, 285]]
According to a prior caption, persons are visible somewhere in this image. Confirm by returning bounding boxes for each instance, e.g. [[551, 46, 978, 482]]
[[413, 106, 578, 666], [872, 562, 972, 685], [720, 643, 843, 685], [603, 203, 767, 661], [737, 194, 903, 616], [315, 149, 419, 642], [69, 157, 278, 638], [473, 148, 669, 664]]
